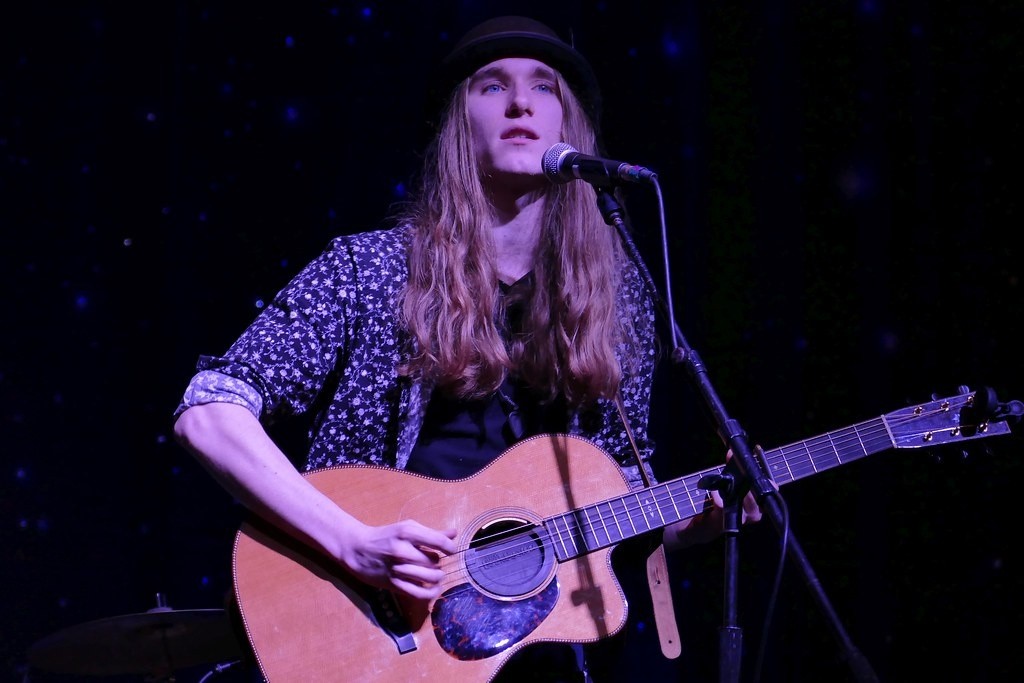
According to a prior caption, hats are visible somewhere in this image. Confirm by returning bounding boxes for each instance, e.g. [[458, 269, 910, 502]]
[[442, 16, 602, 107]]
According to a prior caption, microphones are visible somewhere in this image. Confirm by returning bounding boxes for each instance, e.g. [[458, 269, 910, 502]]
[[542, 144, 638, 189]]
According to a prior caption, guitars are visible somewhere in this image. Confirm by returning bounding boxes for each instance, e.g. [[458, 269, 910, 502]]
[[231, 381, 1024, 683]]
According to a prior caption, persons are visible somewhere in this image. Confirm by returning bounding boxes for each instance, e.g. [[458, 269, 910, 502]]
[[169, 29, 780, 683]]
